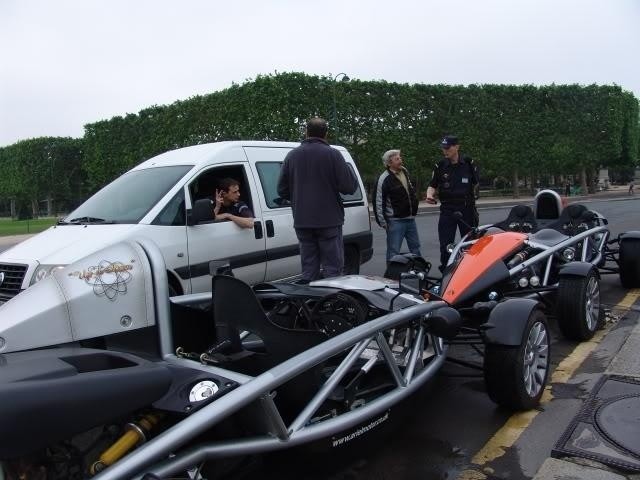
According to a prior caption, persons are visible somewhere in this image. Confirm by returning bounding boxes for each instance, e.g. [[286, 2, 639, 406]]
[[565, 180, 571, 197], [628, 180, 635, 193], [210, 177, 254, 229], [425, 135, 480, 275], [372, 149, 422, 269], [277, 117, 358, 282]]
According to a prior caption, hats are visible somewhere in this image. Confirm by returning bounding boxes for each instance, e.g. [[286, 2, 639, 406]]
[[441, 135, 458, 149]]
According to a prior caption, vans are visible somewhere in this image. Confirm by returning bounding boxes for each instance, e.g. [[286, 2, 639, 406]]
[[0, 140, 374, 304]]
